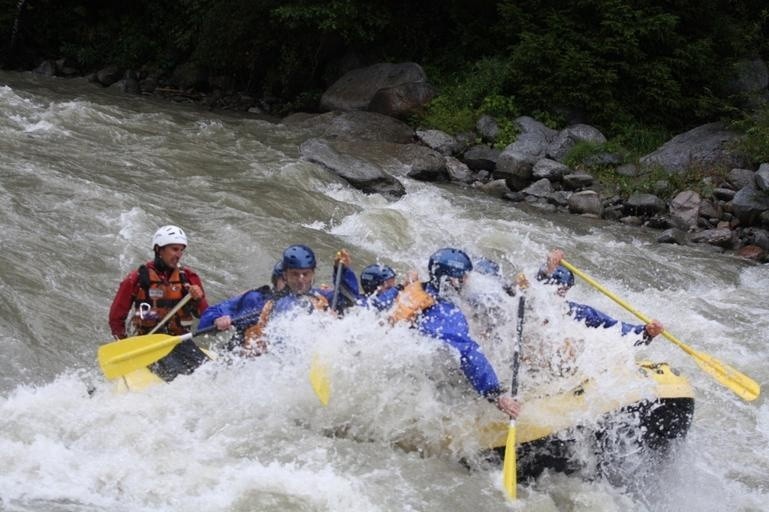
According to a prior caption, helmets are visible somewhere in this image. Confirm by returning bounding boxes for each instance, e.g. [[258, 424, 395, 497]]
[[152, 225, 187, 250], [360, 264, 395, 293], [283, 245, 316, 269], [537, 262, 574, 287], [272, 261, 283, 282], [428, 248, 473, 278], [475, 260, 498, 277]]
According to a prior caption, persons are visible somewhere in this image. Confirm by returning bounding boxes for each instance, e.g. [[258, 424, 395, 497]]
[[357, 249, 526, 418], [536, 248, 664, 350], [108, 225, 214, 383], [198, 244, 357, 356]]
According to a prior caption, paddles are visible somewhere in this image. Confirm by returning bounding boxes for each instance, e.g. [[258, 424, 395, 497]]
[[559, 259, 763, 401], [95, 310, 264, 380], [502, 275, 529, 502], [309, 253, 345, 406]]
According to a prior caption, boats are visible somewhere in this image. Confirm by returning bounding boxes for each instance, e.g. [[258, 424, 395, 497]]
[[116, 348, 695, 490]]
